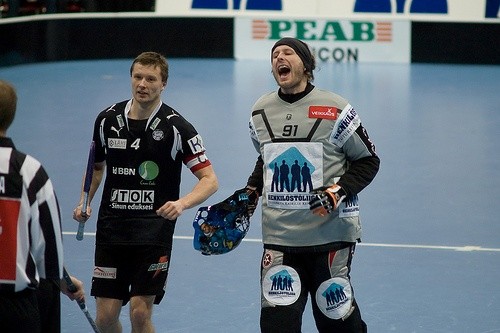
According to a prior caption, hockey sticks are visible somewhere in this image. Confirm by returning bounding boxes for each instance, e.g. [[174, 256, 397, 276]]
[[62, 268, 99, 333], [73, 141, 99, 242]]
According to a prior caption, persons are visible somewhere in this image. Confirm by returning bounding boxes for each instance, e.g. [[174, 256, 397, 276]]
[[235, 37, 380, 333], [73, 52, 218, 333], [0, 78, 85, 333]]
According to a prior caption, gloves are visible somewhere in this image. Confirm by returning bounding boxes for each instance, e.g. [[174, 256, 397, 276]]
[[309, 184, 348, 217], [244, 184, 259, 217]]
